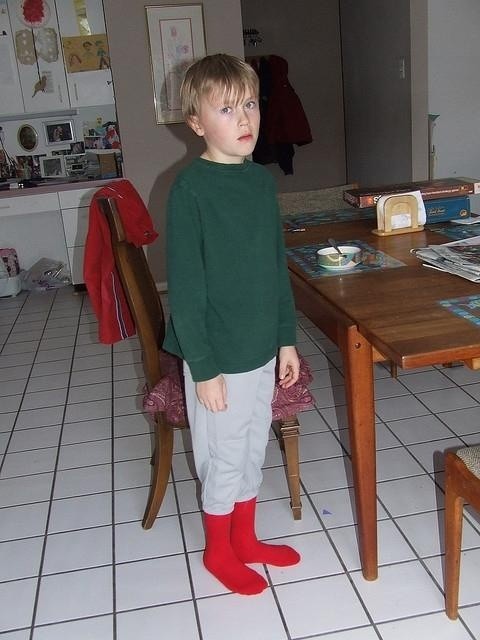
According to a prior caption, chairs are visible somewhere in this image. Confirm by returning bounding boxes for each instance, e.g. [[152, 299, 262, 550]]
[[440, 443, 480, 620], [97, 190, 305, 529]]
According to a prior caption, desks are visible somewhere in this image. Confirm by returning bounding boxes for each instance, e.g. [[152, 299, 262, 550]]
[[0, 177, 121, 297]]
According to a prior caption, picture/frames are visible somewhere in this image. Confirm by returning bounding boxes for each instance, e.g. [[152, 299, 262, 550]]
[[17, 124, 39, 151], [41, 118, 77, 147], [38, 156, 66, 179], [143, 3, 208, 126]]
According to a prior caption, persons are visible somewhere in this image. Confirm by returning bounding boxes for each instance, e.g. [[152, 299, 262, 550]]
[[161, 49, 302, 598], [17, 125, 99, 177]]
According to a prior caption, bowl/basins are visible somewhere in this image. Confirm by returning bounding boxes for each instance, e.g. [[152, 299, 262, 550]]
[[317, 246, 362, 273]]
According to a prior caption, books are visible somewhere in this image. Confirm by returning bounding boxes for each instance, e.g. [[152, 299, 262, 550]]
[[409, 233, 480, 285]]
